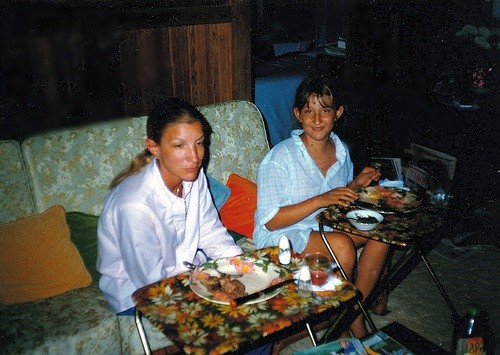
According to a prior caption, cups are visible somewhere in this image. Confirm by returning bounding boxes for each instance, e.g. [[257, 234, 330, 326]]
[[430, 178, 454, 206], [304, 252, 332, 287]]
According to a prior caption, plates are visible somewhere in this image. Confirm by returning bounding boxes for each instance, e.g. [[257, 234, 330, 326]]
[[189, 255, 286, 305], [350, 186, 423, 214]]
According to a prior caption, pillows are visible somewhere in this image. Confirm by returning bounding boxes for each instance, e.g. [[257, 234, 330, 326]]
[[0, 205, 92, 306], [218, 174, 257, 237]]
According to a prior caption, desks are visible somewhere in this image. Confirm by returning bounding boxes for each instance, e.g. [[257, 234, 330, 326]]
[[254, 37, 367, 145]]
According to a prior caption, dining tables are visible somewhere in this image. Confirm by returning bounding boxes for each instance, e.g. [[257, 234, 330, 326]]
[[315, 185, 462, 332], [133, 246, 360, 355]]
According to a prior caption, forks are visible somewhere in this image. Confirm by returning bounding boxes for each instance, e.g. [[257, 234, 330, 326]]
[[183, 261, 243, 278]]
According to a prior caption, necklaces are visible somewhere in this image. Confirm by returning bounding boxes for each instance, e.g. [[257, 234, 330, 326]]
[[176, 189, 179, 193]]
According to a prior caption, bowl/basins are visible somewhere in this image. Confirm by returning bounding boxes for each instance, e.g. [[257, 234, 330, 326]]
[[346, 210, 384, 230], [380, 181, 404, 188]]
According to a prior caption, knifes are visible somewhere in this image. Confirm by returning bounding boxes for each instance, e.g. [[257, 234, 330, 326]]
[[230, 278, 297, 307]]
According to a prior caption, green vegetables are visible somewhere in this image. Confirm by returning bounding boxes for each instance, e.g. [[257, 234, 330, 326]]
[[356, 215, 379, 224]]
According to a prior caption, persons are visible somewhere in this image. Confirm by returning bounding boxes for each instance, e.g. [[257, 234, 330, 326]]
[[253, 76, 389, 338], [94, 98, 276, 355]]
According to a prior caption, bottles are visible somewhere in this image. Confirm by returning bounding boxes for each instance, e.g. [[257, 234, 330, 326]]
[[298, 266, 313, 299], [278, 236, 291, 267], [452, 306, 494, 355]]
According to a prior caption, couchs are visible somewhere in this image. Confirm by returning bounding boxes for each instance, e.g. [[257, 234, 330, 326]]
[[0, 99, 270, 355]]
